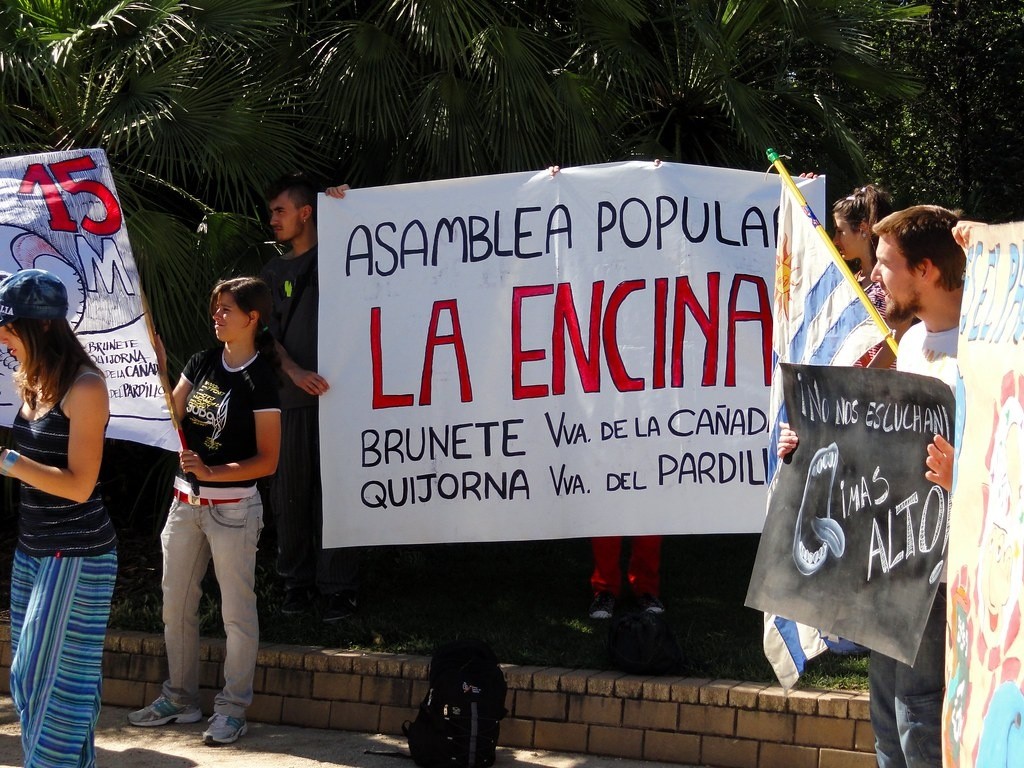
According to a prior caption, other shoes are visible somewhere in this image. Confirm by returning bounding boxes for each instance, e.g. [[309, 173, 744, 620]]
[[322, 592, 354, 624], [824, 637, 871, 655], [281, 586, 316, 614]]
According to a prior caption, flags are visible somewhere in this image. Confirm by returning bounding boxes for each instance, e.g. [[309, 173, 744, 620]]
[[761, 180, 892, 691]]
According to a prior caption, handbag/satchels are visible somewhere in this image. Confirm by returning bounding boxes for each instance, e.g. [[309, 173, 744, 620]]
[[608, 612, 689, 675]]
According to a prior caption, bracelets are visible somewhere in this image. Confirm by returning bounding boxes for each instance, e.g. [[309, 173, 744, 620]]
[[0, 443, 22, 475]]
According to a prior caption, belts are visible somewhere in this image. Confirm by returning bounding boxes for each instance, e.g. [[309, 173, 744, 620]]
[[174, 488, 242, 506]]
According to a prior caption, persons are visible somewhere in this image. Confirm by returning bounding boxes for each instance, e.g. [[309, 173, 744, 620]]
[[127, 277, 281, 744], [545, 160, 664, 620], [268, 176, 351, 622], [0, 268, 117, 768], [832, 185, 912, 371], [778, 205, 967, 768]]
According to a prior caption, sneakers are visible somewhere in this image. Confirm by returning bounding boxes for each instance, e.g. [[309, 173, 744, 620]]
[[203, 711, 248, 744], [127, 690, 202, 726], [588, 591, 615, 619], [637, 595, 665, 614]]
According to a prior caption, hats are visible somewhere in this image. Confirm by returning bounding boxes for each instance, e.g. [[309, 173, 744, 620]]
[[0, 271, 68, 326]]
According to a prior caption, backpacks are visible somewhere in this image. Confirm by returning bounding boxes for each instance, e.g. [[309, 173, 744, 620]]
[[402, 636, 509, 768]]
